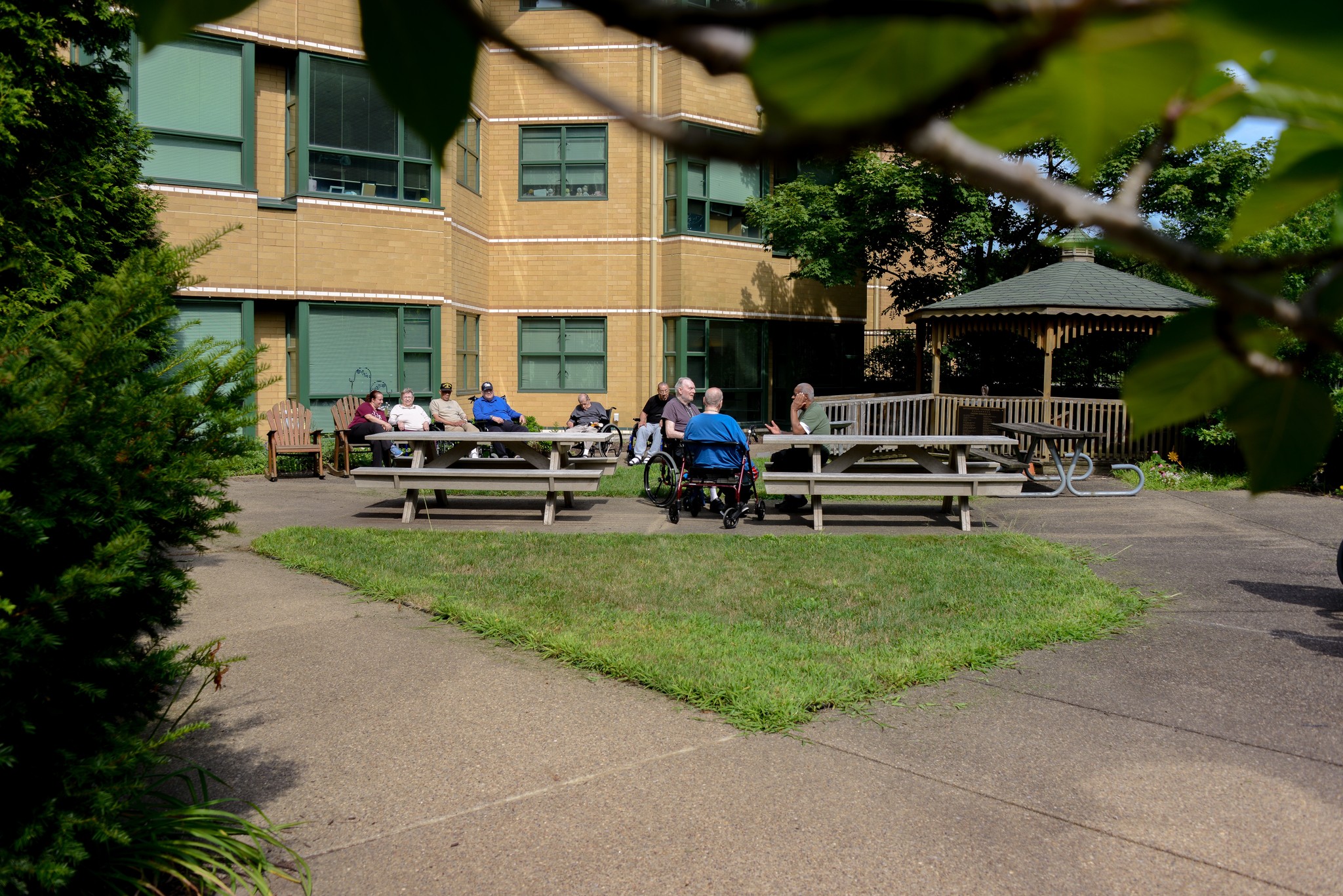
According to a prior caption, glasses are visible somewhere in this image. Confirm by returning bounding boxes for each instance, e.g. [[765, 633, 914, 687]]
[[402, 396, 413, 400], [484, 390, 494, 393], [658, 389, 669, 394]]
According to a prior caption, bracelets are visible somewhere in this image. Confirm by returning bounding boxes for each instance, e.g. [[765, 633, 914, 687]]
[[780, 430, 783, 435], [464, 419, 467, 422]]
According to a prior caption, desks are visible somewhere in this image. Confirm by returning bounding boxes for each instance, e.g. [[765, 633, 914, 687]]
[[763, 434, 1018, 531], [365, 431, 614, 525], [991, 422, 1106, 495]]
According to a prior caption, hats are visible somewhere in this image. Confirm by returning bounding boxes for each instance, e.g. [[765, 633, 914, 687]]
[[588, 422, 603, 429], [482, 382, 493, 391], [440, 383, 453, 392]]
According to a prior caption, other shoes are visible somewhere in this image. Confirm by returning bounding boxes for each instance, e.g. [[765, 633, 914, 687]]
[[513, 455, 521, 458], [739, 501, 749, 518], [775, 495, 808, 512]]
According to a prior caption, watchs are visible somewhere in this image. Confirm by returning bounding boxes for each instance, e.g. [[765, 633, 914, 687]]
[[490, 415, 493, 420]]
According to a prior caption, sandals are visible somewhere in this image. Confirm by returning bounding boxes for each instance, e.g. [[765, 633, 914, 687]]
[[581, 455, 588, 458], [628, 456, 644, 466], [643, 456, 651, 465]]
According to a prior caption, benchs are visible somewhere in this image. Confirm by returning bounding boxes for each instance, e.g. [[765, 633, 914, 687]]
[[764, 447, 1029, 495], [350, 456, 618, 491]]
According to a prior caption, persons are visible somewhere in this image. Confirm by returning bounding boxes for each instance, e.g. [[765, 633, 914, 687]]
[[662, 377, 725, 513], [430, 383, 480, 458], [685, 387, 759, 516], [765, 383, 830, 510], [628, 382, 674, 465], [473, 382, 527, 458], [565, 393, 609, 458], [388, 388, 437, 457], [347, 390, 404, 468]]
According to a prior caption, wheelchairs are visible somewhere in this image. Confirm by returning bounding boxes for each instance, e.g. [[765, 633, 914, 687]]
[[626, 418, 668, 466], [644, 428, 722, 508], [563, 407, 623, 457], [467, 395, 520, 458], [669, 424, 765, 529], [429, 420, 484, 458], [392, 423, 445, 457]]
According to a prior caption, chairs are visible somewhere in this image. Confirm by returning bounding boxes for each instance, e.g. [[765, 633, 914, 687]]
[[326, 395, 389, 476], [264, 400, 326, 480]]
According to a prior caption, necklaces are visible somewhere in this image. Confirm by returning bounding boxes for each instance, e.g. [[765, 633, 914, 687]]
[[676, 397, 693, 419], [704, 410, 718, 413]]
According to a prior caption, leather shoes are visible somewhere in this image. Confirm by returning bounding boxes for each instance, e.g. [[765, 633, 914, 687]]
[[709, 498, 725, 514]]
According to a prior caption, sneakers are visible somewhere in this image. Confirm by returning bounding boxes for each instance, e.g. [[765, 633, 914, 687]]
[[390, 445, 404, 457], [470, 452, 479, 458]]
